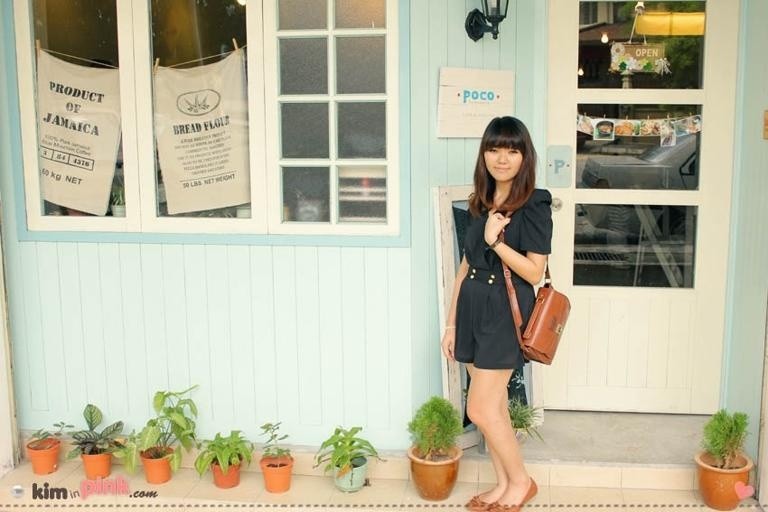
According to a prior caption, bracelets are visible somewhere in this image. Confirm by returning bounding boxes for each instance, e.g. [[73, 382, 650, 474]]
[[444, 325, 457, 331], [484, 238, 503, 251]]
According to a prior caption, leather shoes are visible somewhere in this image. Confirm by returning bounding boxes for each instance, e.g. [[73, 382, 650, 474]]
[[465, 474, 538, 512]]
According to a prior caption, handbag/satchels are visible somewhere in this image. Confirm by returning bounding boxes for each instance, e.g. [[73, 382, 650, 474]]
[[519, 284, 572, 367]]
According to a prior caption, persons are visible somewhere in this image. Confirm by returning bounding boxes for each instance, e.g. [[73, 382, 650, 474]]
[[440, 115, 554, 508]]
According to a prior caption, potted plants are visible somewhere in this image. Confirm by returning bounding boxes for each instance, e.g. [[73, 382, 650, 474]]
[[695, 408, 754, 511], [24, 385, 464, 501]]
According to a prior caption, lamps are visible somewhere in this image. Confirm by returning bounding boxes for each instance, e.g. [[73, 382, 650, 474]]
[[466, 1, 510, 45]]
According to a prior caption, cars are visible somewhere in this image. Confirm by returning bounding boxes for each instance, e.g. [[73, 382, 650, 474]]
[[576, 130, 698, 244]]
